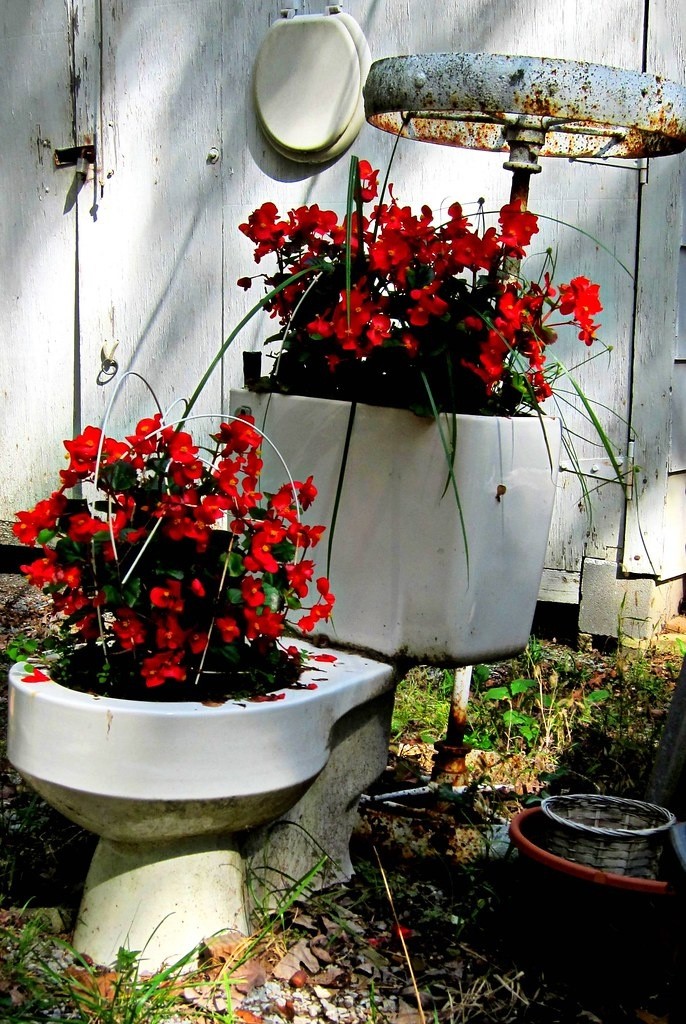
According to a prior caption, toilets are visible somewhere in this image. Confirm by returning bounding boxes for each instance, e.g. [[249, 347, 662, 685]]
[[5, 387, 563, 990]]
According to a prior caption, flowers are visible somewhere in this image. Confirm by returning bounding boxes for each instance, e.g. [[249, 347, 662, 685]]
[[228, 161, 658, 582], [6, 261, 343, 701]]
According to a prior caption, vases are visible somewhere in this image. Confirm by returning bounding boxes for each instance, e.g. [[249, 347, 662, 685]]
[[509, 794, 684, 906]]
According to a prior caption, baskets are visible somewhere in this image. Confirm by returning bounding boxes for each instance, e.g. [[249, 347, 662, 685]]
[[542, 795, 676, 880]]
